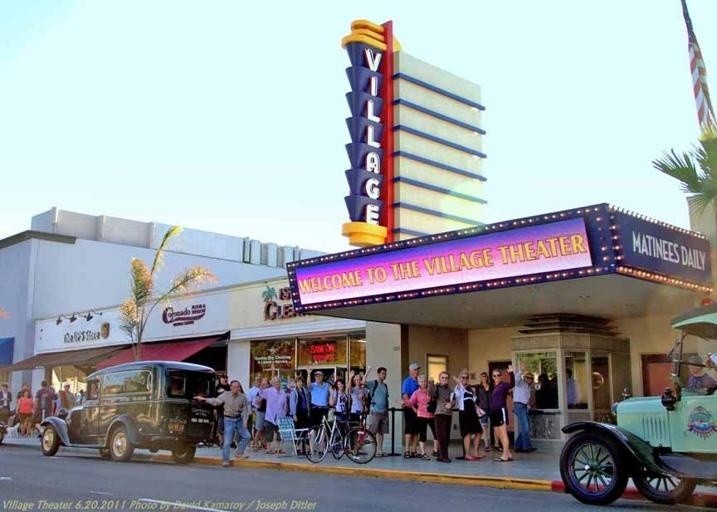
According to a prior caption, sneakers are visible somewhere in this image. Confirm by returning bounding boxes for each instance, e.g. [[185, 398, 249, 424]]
[[223, 461, 230, 466], [236, 451, 249, 458]]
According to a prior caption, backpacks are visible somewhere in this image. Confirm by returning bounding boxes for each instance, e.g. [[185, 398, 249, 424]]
[[368, 380, 387, 406]]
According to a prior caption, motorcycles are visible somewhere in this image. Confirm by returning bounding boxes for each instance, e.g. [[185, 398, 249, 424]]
[[0, 398, 17, 442]]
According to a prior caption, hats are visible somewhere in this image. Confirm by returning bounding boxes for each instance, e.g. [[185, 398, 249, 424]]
[[409, 364, 420, 369], [315, 371, 323, 376]]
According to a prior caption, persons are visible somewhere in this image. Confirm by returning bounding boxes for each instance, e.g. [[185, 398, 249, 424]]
[[428, 372, 454, 463], [409, 375, 439, 460], [401, 364, 424, 458], [475, 372, 493, 452], [489, 365, 515, 462], [455, 370, 486, 460], [0, 381, 74, 445], [194, 367, 388, 468], [512, 364, 537, 452], [536, 367, 580, 408], [687, 357, 716, 394]]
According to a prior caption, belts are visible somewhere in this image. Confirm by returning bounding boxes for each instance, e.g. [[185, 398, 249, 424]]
[[225, 415, 241, 418]]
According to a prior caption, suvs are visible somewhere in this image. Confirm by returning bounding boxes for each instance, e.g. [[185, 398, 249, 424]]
[[559, 306, 717, 504], [39, 361, 220, 461]]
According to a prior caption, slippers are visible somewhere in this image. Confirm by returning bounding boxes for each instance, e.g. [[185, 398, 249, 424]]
[[466, 447, 537, 461], [263, 445, 285, 454], [376, 452, 388, 456]]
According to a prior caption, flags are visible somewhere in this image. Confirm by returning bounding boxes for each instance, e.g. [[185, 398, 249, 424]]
[[682, 2, 715, 133]]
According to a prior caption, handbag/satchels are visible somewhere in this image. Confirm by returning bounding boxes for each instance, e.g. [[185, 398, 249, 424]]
[[476, 405, 485, 418], [427, 385, 438, 413], [365, 404, 369, 413]]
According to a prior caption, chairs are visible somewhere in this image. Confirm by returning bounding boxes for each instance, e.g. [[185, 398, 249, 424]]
[[276, 416, 308, 458]]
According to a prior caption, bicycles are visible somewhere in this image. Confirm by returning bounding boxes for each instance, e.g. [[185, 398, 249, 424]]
[[303, 403, 377, 462]]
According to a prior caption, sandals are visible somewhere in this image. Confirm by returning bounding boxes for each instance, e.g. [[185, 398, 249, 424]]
[[404, 450, 450, 463]]
